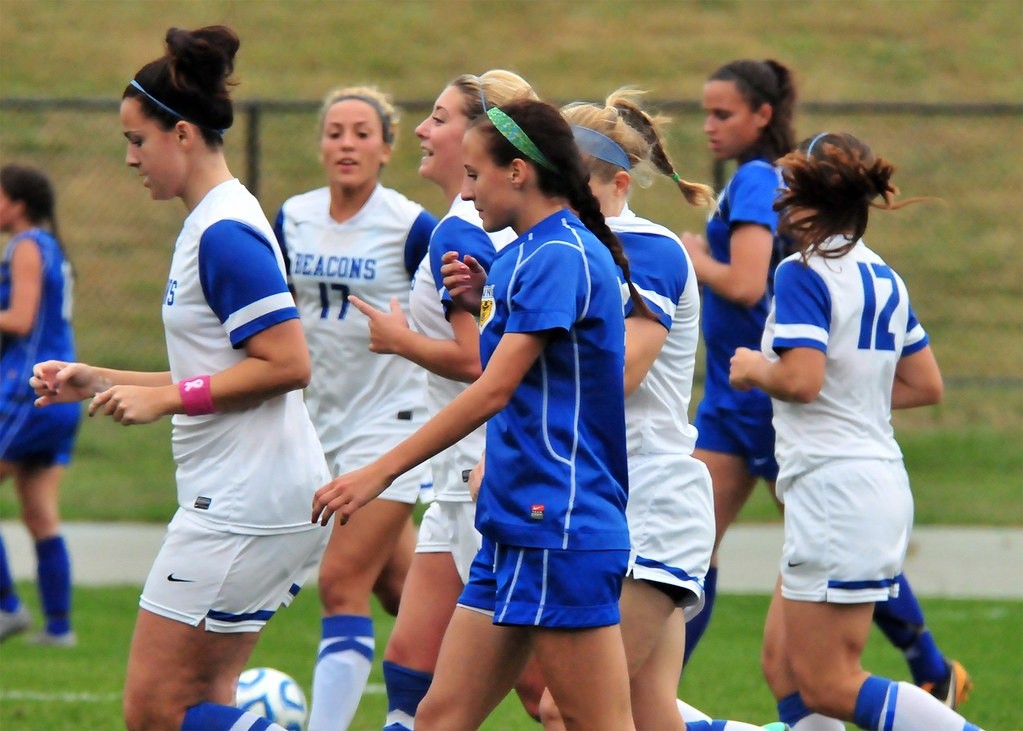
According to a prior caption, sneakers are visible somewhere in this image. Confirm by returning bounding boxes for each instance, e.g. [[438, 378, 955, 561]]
[[914, 659, 972, 707]]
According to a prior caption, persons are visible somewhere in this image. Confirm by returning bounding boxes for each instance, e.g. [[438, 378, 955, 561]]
[[0, 162, 82, 646], [29, 25, 336, 731], [273, 58, 987, 731]]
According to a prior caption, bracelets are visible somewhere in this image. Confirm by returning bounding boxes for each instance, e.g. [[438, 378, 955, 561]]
[[179, 374, 216, 416]]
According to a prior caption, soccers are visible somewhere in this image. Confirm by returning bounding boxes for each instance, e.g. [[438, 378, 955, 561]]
[[235, 666, 309, 731]]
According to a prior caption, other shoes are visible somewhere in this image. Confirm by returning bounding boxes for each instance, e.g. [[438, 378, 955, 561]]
[[0, 601, 31, 640], [24, 631, 78, 648]]
